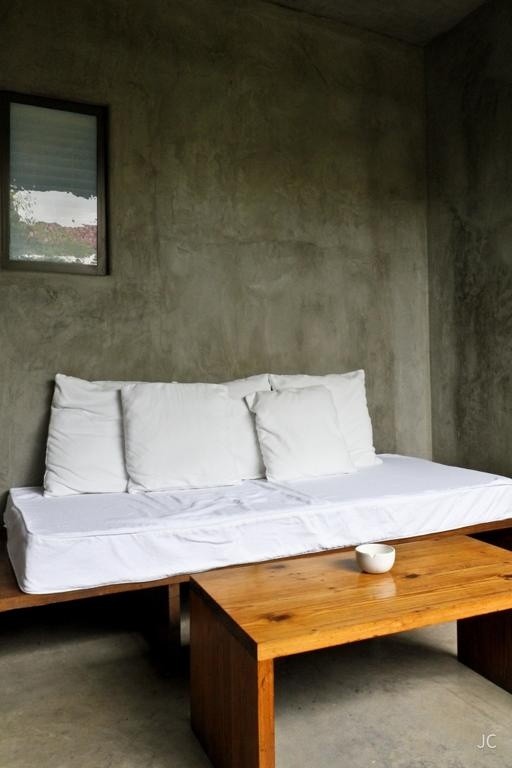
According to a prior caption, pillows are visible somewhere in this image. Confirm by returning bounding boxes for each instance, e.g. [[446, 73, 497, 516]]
[[44, 370, 379, 492]]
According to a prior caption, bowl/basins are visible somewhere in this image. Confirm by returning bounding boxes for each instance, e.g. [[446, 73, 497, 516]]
[[354, 543, 395, 575]]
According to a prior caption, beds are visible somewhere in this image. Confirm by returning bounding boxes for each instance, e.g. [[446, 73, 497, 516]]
[[2, 452, 511, 670]]
